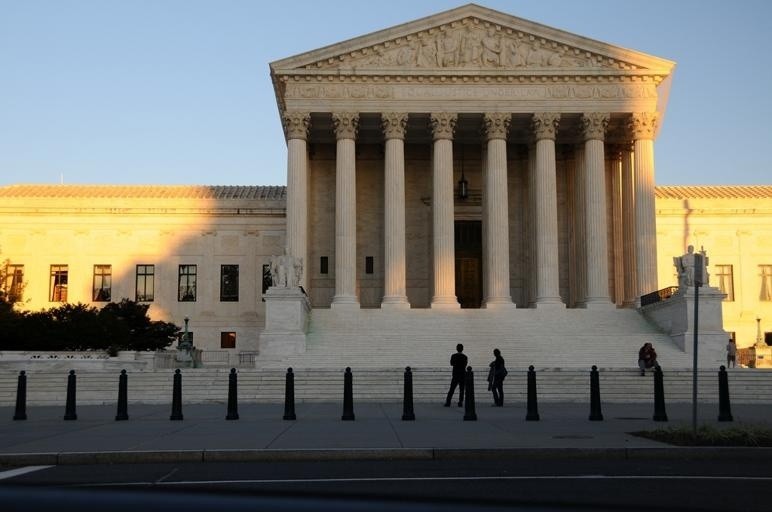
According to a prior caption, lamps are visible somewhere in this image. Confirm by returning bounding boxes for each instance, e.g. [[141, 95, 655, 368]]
[[457, 135, 468, 201]]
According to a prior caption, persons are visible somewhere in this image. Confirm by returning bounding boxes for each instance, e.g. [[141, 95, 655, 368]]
[[488, 348, 508, 407], [672, 245, 712, 286], [637, 342, 663, 377], [726, 338, 737, 368], [442, 343, 468, 407], [269, 245, 304, 288]]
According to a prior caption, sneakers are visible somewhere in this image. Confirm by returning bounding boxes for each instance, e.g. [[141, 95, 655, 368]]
[[443, 402, 463, 407]]
